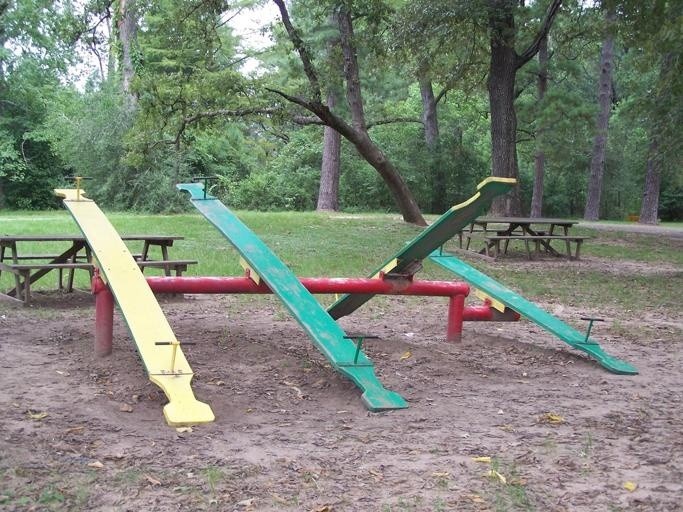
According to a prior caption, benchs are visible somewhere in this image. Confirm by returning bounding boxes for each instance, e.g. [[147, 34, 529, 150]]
[[457, 230, 547, 249], [484, 235, 590, 262], [0, 256, 148, 261], [8, 260, 197, 303]]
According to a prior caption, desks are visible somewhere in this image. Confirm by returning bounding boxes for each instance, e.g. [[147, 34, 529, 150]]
[[0, 236, 184, 307], [446, 218, 591, 262]]
